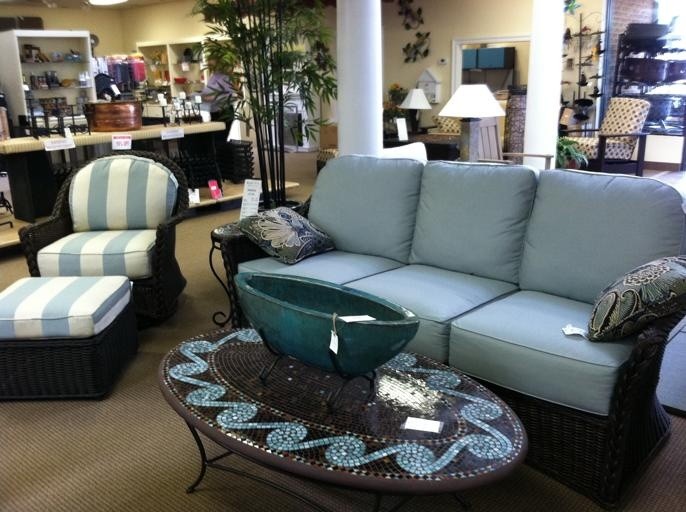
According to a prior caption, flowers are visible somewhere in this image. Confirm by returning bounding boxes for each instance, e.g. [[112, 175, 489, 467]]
[[383, 82, 411, 139]]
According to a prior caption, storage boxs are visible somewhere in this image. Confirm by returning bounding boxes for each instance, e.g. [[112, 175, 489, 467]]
[[461, 47, 516, 69]]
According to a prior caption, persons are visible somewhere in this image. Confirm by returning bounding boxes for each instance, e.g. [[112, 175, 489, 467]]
[[195, 65, 239, 141]]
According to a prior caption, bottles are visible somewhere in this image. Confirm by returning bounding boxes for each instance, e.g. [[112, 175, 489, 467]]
[[22, 70, 93, 91]]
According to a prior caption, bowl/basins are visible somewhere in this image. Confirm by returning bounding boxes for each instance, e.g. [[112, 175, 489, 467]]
[[233, 272, 418, 377], [624, 22, 686, 127], [81, 102, 142, 133], [174, 78, 187, 85]]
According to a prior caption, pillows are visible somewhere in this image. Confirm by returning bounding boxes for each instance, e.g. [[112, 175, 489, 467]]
[[587, 253, 685, 342], [432, 114, 462, 133], [235, 204, 335, 265]]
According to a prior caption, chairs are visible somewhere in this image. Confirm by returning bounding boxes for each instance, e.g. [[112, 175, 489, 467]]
[[478, 119, 555, 170], [18, 151, 190, 332], [427, 99, 507, 150], [558, 97, 652, 176]]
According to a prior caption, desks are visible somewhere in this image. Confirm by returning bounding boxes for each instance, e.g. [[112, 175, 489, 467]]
[[0, 120, 226, 226]]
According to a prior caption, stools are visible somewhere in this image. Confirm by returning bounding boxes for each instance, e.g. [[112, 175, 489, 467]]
[[0, 275, 141, 400], [316, 148, 338, 176]]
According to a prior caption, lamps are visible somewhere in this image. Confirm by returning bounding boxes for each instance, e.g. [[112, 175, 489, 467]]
[[436, 85, 507, 163], [398, 88, 432, 137]]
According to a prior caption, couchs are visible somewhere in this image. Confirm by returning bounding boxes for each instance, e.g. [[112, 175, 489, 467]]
[[220, 156, 685, 512]]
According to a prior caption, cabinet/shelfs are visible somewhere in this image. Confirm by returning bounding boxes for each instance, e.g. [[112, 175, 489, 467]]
[[0, 29, 97, 135], [612, 33, 685, 172], [135, 32, 255, 141], [560, 13, 606, 129]]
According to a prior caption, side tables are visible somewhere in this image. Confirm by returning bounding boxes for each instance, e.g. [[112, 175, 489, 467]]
[[208, 220, 242, 329]]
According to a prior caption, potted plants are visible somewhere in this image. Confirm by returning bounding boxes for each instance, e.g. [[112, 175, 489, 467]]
[[187, 1, 337, 214]]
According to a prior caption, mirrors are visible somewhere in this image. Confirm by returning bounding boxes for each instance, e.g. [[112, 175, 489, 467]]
[[450, 35, 530, 100]]
